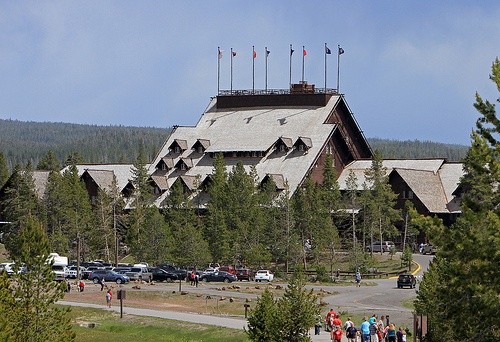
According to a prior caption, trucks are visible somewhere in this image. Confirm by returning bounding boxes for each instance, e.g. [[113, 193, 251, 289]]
[[37, 253, 68, 282]]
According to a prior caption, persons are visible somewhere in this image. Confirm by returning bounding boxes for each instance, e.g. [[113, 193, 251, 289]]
[[355, 269, 362, 287], [64, 276, 113, 308], [190, 271, 200, 287], [315, 308, 407, 342]]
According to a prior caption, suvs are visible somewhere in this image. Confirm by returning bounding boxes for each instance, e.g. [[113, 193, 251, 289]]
[[397, 273, 417, 288], [237, 268, 255, 281], [366, 241, 395, 253]]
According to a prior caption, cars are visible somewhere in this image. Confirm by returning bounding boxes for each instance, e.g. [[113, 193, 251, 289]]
[[421, 244, 438, 255], [255, 269, 274, 281], [65, 260, 193, 284], [0, 262, 27, 275], [196, 263, 238, 283]]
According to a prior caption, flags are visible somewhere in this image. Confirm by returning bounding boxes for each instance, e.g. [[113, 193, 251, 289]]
[[266, 49, 270, 56], [326, 47, 331, 54], [338, 47, 345, 55], [290, 48, 294, 55], [218, 48, 223, 59], [253, 50, 256, 58], [232, 50, 236, 57], [304, 48, 306, 56]]
